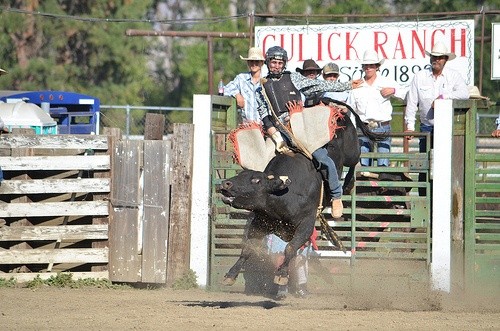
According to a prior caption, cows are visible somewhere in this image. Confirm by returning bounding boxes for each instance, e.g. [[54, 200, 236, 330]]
[[210, 94, 389, 289]]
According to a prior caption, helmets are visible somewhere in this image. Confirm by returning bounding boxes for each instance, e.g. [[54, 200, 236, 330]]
[[263, 46, 288, 64]]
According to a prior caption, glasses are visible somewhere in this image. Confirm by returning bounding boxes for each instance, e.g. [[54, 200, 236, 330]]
[[328, 75, 336, 77], [432, 56, 445, 59]]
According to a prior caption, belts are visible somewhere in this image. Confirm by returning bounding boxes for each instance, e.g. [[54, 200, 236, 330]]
[[365, 121, 391, 129]]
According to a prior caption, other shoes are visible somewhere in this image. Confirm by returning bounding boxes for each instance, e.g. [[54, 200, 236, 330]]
[[276, 286, 289, 299], [295, 286, 312, 298], [331, 199, 343, 218]]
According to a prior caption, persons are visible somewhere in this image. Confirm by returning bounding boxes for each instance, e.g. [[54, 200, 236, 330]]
[[349, 49, 407, 168], [296, 57, 322, 79], [224, 47, 266, 126], [241, 210, 314, 298], [404, 43, 469, 197], [255, 46, 365, 218], [323, 62, 349, 102]]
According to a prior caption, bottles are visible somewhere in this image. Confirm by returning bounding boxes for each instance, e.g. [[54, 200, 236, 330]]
[[217, 79, 224, 96]]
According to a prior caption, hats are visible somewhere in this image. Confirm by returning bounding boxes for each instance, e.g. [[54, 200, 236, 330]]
[[239, 47, 265, 60], [469, 86, 489, 100], [360, 49, 385, 65], [424, 42, 457, 61], [296, 59, 323, 72], [323, 62, 339, 74]]
